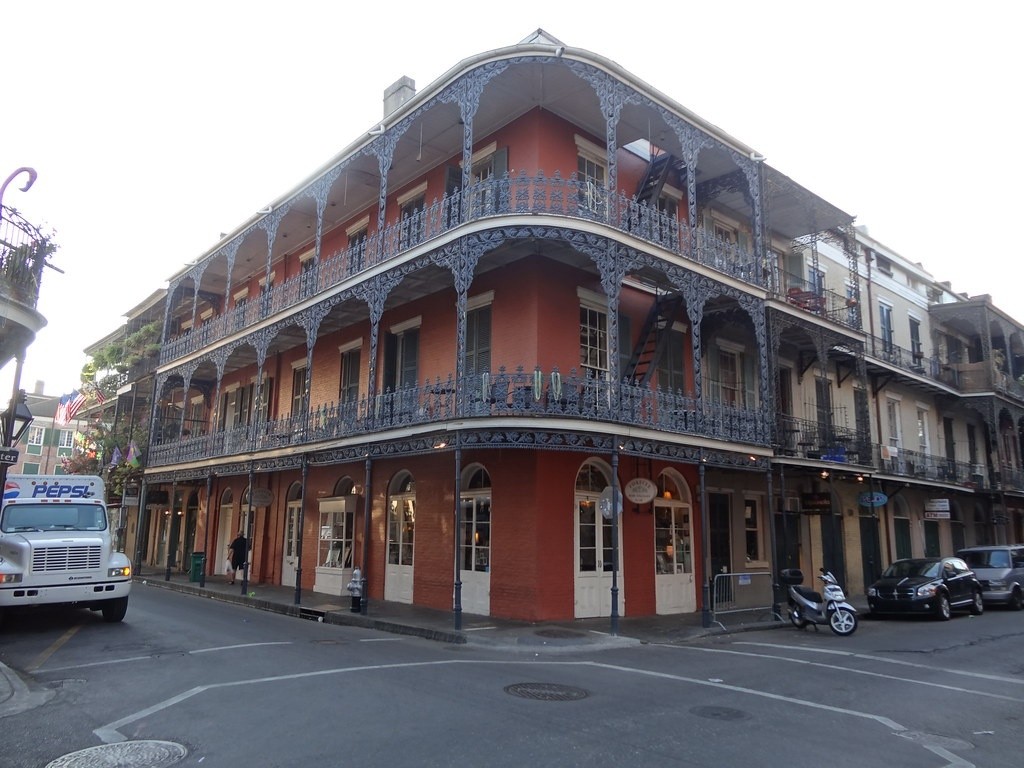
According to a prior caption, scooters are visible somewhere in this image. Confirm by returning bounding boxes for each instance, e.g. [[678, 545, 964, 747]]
[[782, 566, 859, 636]]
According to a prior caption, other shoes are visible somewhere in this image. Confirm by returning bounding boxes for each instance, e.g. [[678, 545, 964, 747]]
[[229, 581, 234, 585]]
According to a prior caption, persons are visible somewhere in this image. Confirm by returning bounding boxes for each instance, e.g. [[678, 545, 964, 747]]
[[225, 530, 251, 586]]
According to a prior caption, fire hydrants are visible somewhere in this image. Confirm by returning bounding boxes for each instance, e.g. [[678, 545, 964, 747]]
[[346, 571, 365, 613]]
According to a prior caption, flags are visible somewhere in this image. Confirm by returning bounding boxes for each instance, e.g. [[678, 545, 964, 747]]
[[111, 446, 121, 467], [54, 395, 70, 427], [65, 389, 86, 419], [126, 440, 141, 469]]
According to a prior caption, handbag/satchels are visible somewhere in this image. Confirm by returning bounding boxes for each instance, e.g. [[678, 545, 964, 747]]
[[225, 559, 234, 575]]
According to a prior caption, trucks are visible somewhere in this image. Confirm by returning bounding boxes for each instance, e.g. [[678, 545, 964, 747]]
[[0, 475, 132, 624]]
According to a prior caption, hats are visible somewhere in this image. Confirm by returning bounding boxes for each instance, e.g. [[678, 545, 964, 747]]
[[237, 530, 245, 535]]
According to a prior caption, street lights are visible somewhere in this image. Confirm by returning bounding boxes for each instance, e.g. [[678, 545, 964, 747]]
[[0, 388, 34, 512]]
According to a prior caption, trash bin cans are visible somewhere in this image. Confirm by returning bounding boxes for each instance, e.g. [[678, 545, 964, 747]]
[[190, 552, 205, 582]]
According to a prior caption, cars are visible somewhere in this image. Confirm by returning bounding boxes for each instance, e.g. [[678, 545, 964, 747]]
[[868, 558, 985, 618], [955, 545, 1024, 610]]
